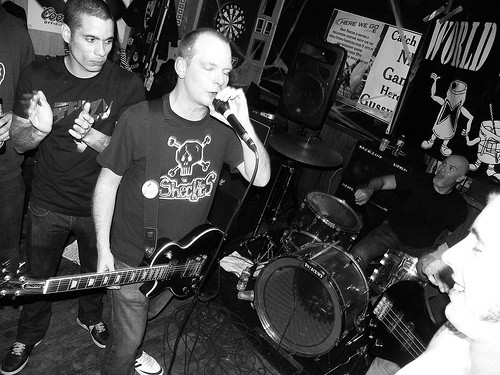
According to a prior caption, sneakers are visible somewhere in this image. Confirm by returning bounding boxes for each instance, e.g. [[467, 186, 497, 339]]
[[0, 338, 43, 375], [76, 317, 109, 349], [134, 350, 164, 375]]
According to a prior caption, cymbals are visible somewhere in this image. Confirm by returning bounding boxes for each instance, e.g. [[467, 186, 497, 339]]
[[252, 110, 345, 168]]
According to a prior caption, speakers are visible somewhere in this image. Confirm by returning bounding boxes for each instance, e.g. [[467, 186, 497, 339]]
[[277, 34, 348, 130], [208, 110, 288, 243]]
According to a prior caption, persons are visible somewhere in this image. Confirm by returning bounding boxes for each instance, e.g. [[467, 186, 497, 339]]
[[395, 193, 500, 375], [92, 27, 271, 375], [350, 155, 470, 274], [0, 0, 147, 375], [366, 210, 485, 375]]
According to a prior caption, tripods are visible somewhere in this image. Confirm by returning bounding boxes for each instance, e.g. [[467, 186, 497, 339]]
[[225, 158, 298, 262]]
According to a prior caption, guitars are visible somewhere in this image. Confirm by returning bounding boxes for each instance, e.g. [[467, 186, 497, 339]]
[[0, 223, 230, 302], [369, 294, 426, 357]]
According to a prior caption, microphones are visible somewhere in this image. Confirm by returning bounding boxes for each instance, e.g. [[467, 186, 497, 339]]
[[212, 98, 258, 153]]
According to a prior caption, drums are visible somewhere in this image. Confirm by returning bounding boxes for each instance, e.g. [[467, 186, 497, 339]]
[[277, 190, 363, 256], [255, 242, 369, 360]]
